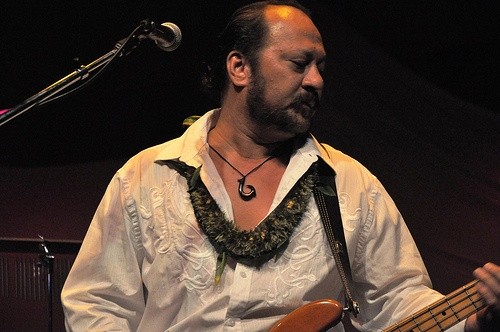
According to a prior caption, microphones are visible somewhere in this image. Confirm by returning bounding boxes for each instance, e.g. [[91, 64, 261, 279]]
[[142, 20, 182, 52]]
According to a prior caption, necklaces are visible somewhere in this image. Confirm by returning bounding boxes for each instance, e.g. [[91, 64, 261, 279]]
[[208, 143, 286, 200]]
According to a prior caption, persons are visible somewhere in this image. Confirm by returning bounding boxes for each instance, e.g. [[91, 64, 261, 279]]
[[60, 0, 500, 332]]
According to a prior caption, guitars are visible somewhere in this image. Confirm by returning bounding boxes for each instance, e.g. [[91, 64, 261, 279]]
[[267, 268, 500, 332]]
